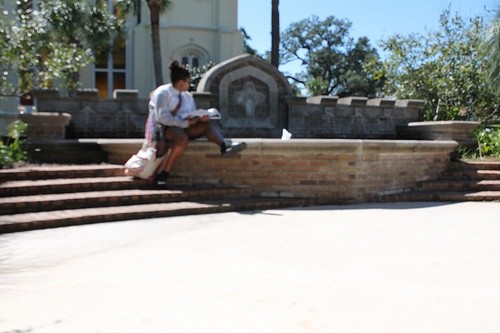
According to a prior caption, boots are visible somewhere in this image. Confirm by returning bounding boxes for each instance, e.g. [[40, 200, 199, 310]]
[[154, 164, 175, 189], [219, 139, 246, 158]]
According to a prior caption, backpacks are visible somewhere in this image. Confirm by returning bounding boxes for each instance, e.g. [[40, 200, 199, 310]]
[[124, 146, 165, 184]]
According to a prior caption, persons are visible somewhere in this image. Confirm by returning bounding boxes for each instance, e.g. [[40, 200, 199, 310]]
[[140, 61, 246, 182]]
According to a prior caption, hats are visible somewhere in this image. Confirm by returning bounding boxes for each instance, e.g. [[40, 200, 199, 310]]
[[170, 63, 188, 83]]
